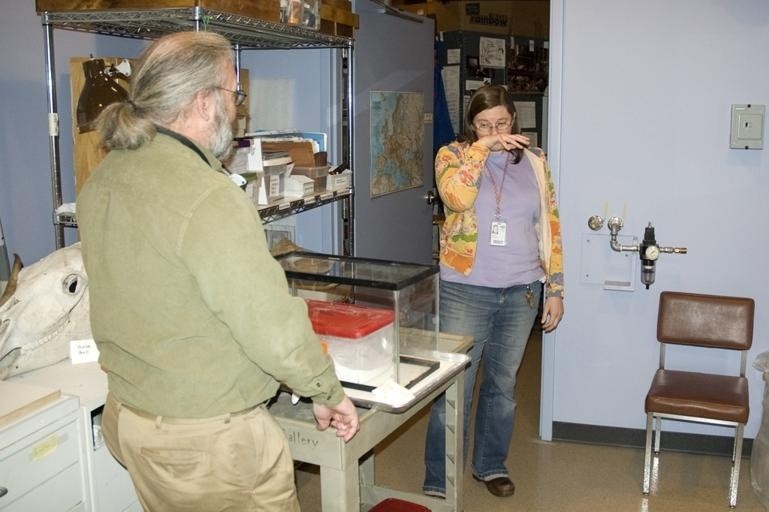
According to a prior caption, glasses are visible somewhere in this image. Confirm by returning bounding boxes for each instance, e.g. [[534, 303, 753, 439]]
[[471, 113, 514, 134], [211, 83, 249, 107]]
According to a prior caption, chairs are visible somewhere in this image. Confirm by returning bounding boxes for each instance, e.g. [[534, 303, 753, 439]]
[[642, 290, 754, 509]]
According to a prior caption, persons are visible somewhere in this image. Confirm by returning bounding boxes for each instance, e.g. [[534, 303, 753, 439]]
[[421, 82, 565, 501], [73, 28, 361, 512]]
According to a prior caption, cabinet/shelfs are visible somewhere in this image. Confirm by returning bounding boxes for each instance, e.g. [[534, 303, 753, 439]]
[[41, 5, 357, 308], [0, 357, 146, 512]]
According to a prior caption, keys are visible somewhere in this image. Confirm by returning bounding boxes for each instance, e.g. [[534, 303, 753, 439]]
[[526, 290, 536, 309]]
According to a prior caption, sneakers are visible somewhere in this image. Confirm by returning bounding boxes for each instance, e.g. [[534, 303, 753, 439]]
[[472, 471, 515, 497]]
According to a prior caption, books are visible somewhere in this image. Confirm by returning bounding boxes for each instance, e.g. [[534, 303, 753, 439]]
[[245, 124, 329, 208]]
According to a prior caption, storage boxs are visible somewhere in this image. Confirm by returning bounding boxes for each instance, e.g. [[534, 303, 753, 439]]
[[427, 0, 512, 34]]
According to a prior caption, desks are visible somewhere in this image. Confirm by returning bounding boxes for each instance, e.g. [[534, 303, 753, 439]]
[[267, 347, 473, 511]]
